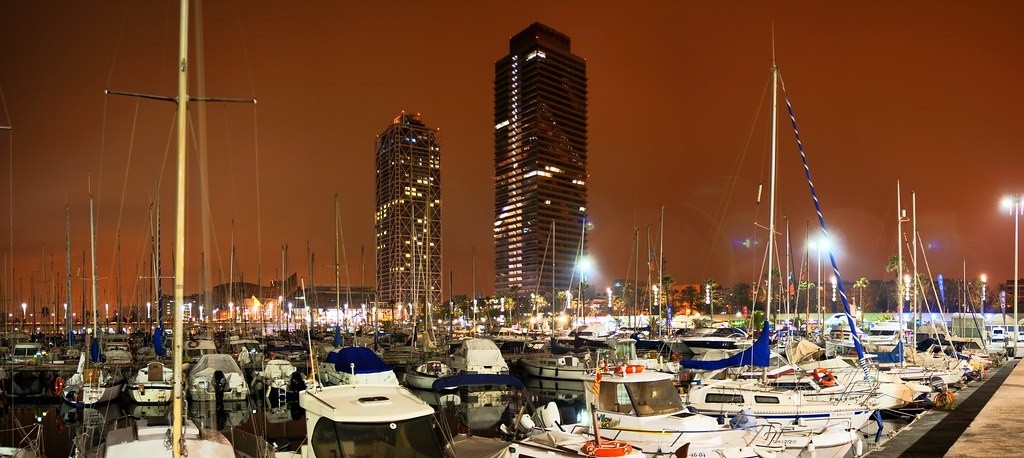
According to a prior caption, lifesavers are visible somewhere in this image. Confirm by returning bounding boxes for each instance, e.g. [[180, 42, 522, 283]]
[[55, 377, 63, 395], [582, 439, 632, 457], [673, 352, 682, 361], [188, 340, 200, 348], [617, 364, 644, 372], [814, 368, 835, 386]]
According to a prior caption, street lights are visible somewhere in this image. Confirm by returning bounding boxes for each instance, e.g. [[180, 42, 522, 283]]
[[1000, 191, 1024, 357]]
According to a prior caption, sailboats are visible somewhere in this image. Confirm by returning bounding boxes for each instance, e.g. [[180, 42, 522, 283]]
[[0, 1, 1024, 458]]
